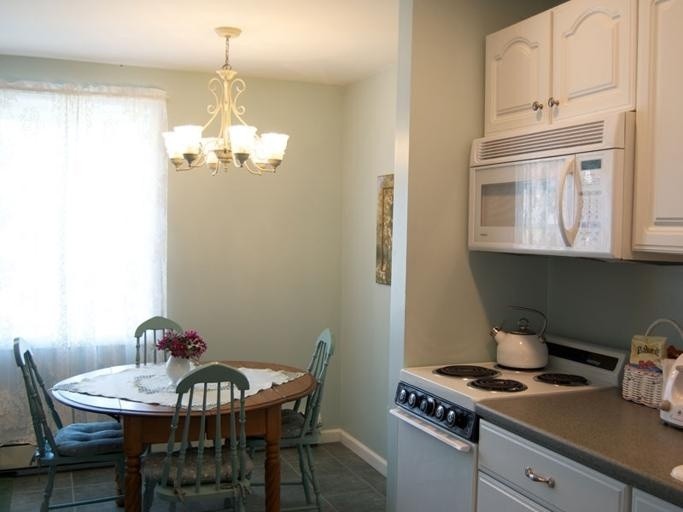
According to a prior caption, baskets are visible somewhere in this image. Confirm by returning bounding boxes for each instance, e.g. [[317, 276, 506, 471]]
[[622, 318, 683, 410]]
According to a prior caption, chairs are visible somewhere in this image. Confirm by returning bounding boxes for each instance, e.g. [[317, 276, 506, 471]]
[[228, 327, 335, 511], [133, 317, 187, 366], [141, 361, 253, 511], [12, 336, 127, 512]]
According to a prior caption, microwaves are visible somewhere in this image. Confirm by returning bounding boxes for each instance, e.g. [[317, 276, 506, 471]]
[[468, 110, 636, 265]]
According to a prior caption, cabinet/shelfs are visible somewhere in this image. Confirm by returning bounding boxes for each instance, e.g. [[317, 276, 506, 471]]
[[475, 397, 682, 512], [633, 0, 683, 258], [482, 0, 637, 136]]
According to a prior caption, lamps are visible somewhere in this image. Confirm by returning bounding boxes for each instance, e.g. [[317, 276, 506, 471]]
[[161, 26, 290, 177]]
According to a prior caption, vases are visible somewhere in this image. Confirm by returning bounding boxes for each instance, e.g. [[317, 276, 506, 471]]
[[167, 355, 191, 384]]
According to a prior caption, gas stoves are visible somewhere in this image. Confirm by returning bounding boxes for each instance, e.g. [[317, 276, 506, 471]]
[[391, 361, 612, 443]]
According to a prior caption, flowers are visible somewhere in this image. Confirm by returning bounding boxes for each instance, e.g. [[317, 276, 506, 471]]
[[156, 329, 207, 366]]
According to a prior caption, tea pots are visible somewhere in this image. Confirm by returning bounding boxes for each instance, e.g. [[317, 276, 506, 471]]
[[488, 305, 550, 371]]
